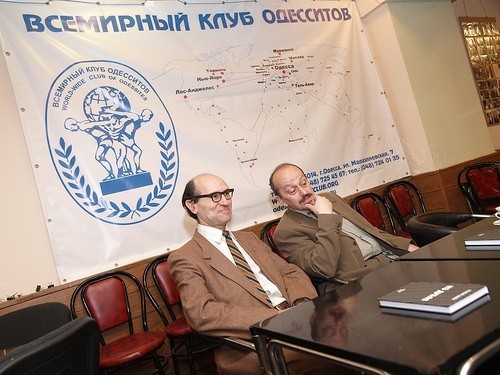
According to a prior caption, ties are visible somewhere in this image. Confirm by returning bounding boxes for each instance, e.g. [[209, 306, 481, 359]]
[[223, 231, 272, 304]]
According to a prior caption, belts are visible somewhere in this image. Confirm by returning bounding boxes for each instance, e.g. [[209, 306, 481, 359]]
[[274, 301, 289, 311]]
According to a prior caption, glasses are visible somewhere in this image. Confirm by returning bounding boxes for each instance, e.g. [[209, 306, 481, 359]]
[[185, 188, 234, 203]]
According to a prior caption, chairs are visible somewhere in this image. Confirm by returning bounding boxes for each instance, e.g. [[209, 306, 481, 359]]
[[354, 159, 500, 231], [408, 212, 494, 247], [1, 218, 286, 375]]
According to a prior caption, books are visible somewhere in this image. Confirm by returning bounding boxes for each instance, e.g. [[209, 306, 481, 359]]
[[381, 295, 491, 324], [466, 246, 500, 251], [464, 229, 500, 245], [379, 281, 488, 314]]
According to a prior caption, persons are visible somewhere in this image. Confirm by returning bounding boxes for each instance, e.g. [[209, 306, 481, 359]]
[[310, 281, 362, 347], [168, 174, 360, 375], [269, 163, 420, 296]]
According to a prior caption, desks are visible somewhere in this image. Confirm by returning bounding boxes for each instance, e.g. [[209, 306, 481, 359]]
[[248, 261, 499, 375], [396, 211, 500, 261]]
